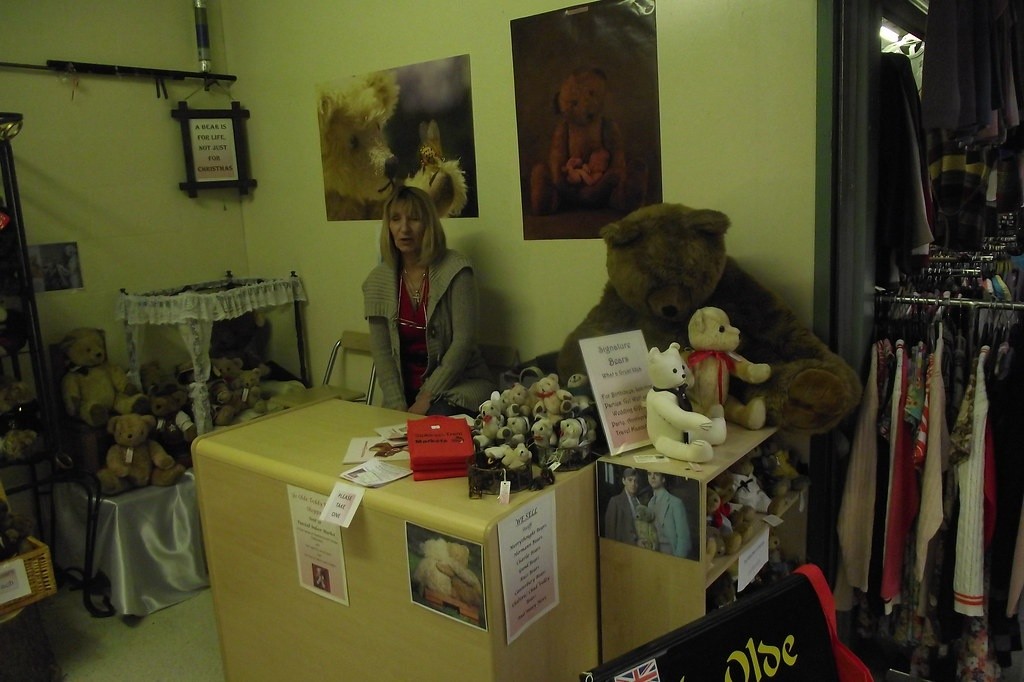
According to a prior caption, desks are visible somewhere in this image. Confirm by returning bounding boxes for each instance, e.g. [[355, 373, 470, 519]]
[[39, 481, 211, 618], [190, 396, 599, 681]]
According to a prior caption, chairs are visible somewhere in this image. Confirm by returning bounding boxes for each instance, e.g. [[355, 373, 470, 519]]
[[269, 331, 378, 409], [580, 566, 873, 682]]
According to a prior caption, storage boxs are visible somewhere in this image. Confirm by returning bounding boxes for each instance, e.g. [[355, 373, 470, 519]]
[[0, 537, 57, 615]]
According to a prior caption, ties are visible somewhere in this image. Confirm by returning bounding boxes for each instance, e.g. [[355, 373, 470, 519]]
[[631, 496, 639, 511]]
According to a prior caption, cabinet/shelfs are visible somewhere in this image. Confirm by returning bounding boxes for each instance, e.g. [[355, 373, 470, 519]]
[[595, 421, 811, 666], [0, 111, 116, 617]]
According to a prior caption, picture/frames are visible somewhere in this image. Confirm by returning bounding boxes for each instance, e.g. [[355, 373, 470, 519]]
[[170, 100, 257, 198]]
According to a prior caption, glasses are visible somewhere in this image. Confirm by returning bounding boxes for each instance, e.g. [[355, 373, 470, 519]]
[[394, 297, 428, 330]]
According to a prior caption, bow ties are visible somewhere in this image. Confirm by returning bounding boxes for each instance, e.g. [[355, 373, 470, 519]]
[[650, 496, 657, 503]]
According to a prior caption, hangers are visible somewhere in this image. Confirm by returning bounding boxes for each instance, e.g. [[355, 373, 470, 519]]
[[889, 33, 922, 54], [871, 211, 1023, 385]]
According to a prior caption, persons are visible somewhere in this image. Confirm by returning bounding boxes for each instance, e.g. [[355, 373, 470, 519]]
[[606, 468, 650, 548], [30, 244, 82, 291], [645, 471, 693, 559], [561, 147, 610, 190], [362, 186, 502, 418]]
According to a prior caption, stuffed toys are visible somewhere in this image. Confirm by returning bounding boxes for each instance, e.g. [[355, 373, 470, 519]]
[[529, 68, 649, 217], [313, 567, 326, 589], [0, 375, 50, 461], [0, 275, 31, 358], [700, 462, 808, 607], [412, 534, 483, 610], [0, 497, 32, 563], [60, 328, 307, 496], [634, 505, 658, 551], [556, 202, 863, 462], [472, 371, 599, 488]]
